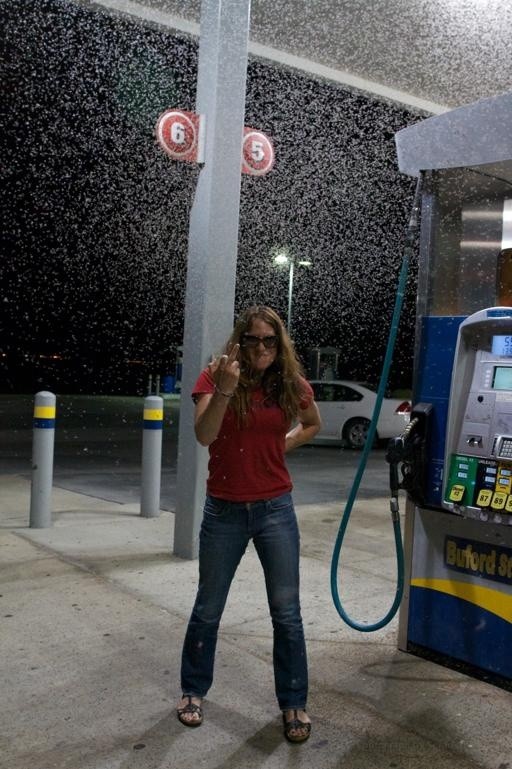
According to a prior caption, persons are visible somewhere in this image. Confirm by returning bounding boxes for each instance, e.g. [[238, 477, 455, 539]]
[[177, 305, 322, 742]]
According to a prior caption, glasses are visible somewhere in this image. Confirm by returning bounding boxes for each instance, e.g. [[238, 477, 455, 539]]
[[242, 333, 278, 349]]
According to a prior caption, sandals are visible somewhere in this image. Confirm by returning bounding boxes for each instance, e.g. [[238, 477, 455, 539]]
[[178, 693, 205, 727], [281, 707, 312, 744]]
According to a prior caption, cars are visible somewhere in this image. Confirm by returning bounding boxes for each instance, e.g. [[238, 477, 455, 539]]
[[289, 378, 412, 452]]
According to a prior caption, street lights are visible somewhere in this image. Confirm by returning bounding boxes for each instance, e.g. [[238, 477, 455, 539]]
[[272, 254, 313, 339]]
